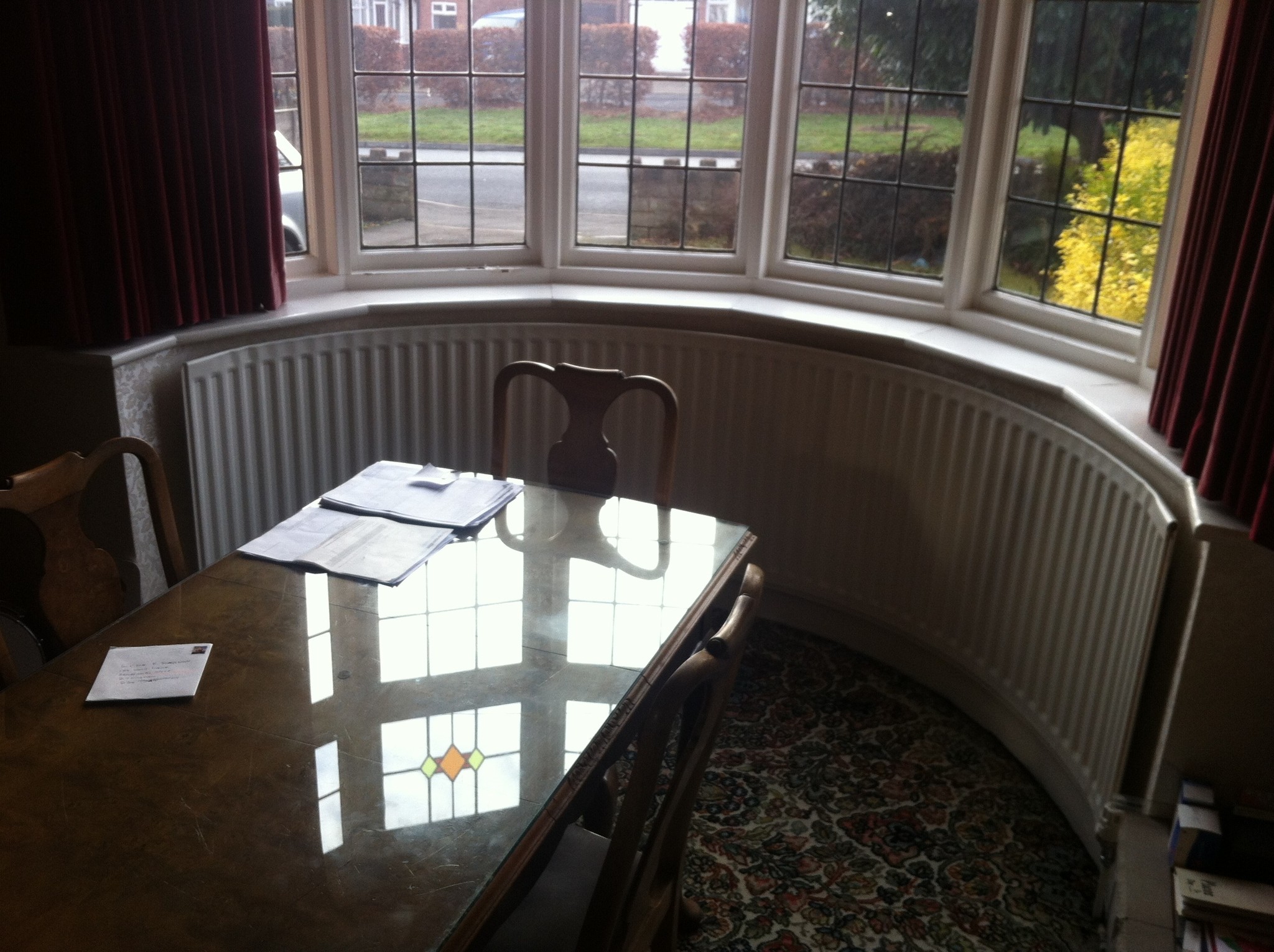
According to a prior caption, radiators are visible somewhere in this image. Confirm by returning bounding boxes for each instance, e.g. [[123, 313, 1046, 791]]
[[178, 322, 1176, 849]]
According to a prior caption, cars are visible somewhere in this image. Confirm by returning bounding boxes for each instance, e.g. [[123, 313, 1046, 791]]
[[474, 8, 525, 31], [274, 128, 305, 253]]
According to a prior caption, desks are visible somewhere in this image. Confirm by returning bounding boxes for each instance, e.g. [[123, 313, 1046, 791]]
[[0, 461, 762, 952]]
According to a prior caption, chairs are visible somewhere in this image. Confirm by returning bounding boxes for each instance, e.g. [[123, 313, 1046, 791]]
[[0, 437, 184, 690], [478, 364, 765, 952]]
